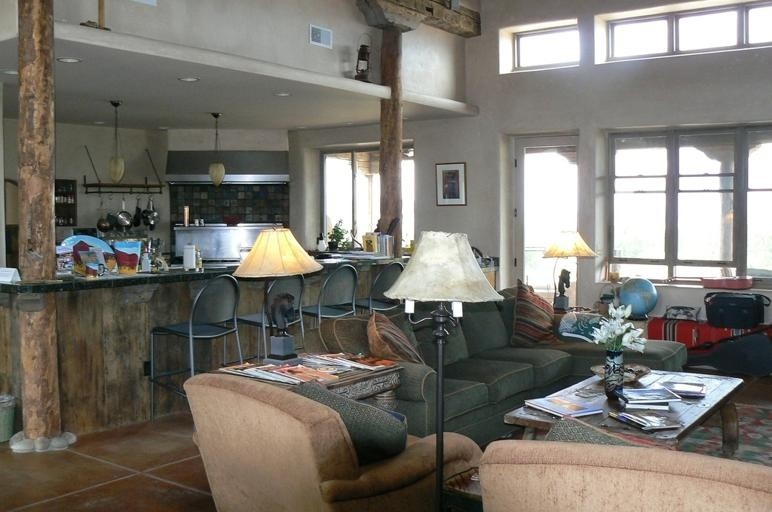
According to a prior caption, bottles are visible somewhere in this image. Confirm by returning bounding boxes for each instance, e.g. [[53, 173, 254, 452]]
[[55, 183, 75, 204], [54, 205, 74, 226], [196, 250, 203, 272], [142, 253, 151, 273]]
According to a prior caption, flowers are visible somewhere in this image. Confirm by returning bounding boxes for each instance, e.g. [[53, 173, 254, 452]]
[[589, 303, 647, 353]]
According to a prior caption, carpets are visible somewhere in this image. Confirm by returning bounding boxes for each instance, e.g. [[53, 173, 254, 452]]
[[676, 400, 772, 466]]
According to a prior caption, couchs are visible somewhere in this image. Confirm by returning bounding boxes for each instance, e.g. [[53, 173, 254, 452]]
[[302, 286, 688, 447]]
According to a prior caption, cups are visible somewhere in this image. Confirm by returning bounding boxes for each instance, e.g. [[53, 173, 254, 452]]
[[85, 262, 105, 280]]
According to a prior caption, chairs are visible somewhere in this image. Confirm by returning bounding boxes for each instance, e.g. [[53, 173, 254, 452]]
[[182, 372, 483, 512], [478, 440, 772, 512]]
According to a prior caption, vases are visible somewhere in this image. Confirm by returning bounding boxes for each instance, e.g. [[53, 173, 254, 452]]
[[605, 350, 624, 399]]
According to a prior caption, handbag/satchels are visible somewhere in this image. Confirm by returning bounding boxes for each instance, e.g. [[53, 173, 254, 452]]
[[704, 292, 771, 328]]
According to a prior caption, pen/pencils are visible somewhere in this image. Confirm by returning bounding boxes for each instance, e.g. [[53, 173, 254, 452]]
[[601, 424, 632, 430]]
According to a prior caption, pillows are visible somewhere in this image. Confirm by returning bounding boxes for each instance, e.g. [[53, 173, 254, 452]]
[[367, 312, 425, 363], [287, 382, 407, 467], [544, 416, 640, 446], [557, 313, 608, 344], [510, 278, 554, 346]]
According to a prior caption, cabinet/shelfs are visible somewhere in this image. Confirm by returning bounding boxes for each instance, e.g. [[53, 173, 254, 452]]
[[55, 179, 77, 226]]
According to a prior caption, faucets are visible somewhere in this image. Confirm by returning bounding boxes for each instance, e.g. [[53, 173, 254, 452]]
[[144, 237, 161, 254]]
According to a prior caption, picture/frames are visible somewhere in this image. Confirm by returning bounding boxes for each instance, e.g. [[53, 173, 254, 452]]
[[435, 163, 466, 206]]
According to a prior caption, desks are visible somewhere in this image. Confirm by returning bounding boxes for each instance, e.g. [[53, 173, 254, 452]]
[[216, 353, 402, 412]]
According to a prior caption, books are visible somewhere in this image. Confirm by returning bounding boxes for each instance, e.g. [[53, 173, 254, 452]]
[[362, 232, 395, 258], [220, 351, 399, 388], [525, 397, 603, 419], [609, 380, 707, 432]]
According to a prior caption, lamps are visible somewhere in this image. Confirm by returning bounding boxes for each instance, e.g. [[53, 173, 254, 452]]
[[355, 33, 374, 84], [383, 231, 504, 512], [232, 228, 323, 359], [542, 231, 598, 313], [207, 113, 225, 188], [108, 101, 126, 187]]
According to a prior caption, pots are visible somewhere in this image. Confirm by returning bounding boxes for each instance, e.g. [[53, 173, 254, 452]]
[[117, 199, 132, 232], [107, 215, 117, 225], [142, 195, 160, 225], [133, 200, 141, 227], [97, 218, 111, 233]]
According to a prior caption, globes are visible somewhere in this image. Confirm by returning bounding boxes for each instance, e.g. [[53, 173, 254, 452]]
[[620, 278, 659, 320]]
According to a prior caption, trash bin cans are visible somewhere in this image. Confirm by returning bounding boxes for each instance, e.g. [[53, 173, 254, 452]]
[[0, 395, 16, 442]]
